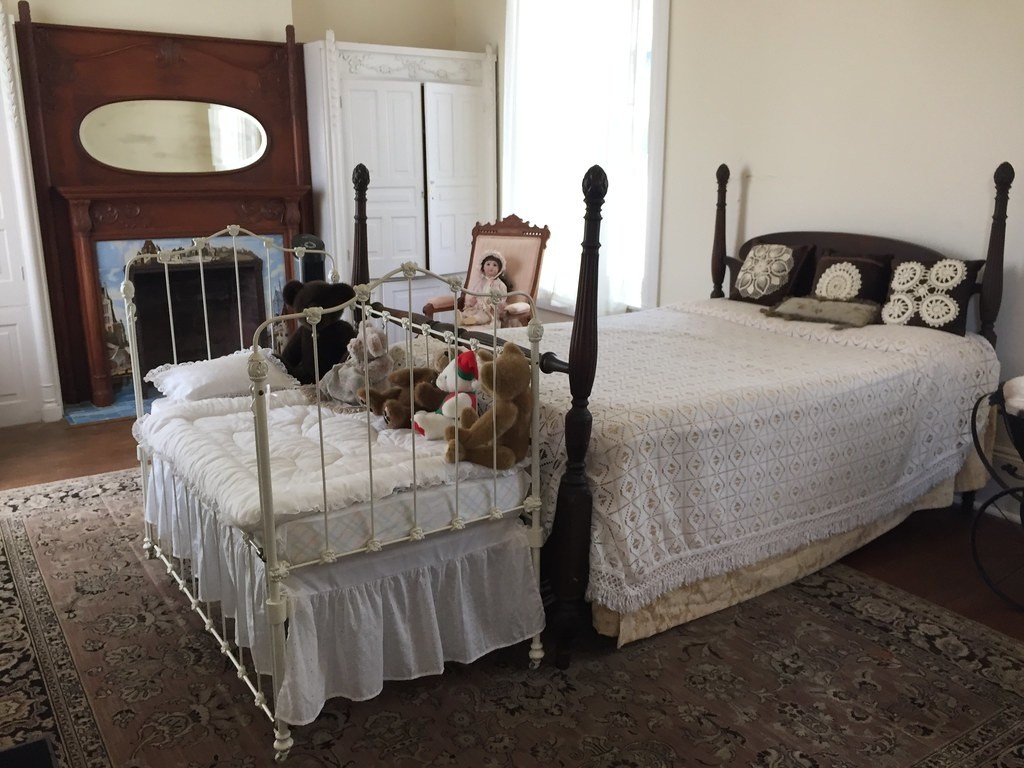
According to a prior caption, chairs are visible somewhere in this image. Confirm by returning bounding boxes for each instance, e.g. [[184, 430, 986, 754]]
[[424, 212, 551, 329]]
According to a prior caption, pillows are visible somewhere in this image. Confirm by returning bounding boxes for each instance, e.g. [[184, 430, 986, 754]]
[[728, 240, 813, 307], [144, 346, 300, 399], [876, 260, 986, 337], [760, 296, 883, 330], [810, 256, 883, 305]]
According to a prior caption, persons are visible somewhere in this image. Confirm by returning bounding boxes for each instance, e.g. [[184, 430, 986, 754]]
[[452, 249, 508, 327]]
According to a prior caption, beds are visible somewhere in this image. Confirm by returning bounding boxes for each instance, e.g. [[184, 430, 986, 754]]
[[353, 161, 1014, 670], [120, 226, 545, 763]]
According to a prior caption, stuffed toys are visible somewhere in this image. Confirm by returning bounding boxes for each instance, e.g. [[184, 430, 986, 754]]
[[445, 342, 533, 469], [319, 320, 395, 407], [355, 347, 465, 429], [410, 349, 479, 441], [270, 280, 357, 386]]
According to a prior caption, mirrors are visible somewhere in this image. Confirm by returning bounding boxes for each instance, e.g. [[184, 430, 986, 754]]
[[73, 96, 272, 178]]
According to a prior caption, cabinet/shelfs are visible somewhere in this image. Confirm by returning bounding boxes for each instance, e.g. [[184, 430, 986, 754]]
[[304, 29, 498, 344]]
